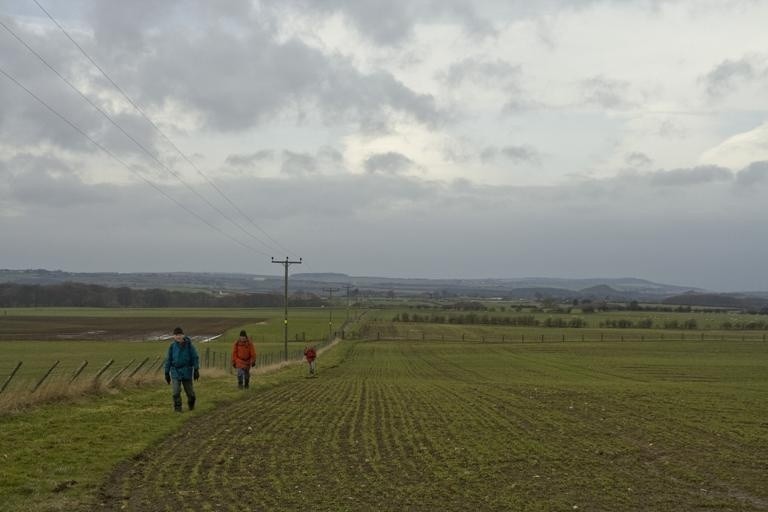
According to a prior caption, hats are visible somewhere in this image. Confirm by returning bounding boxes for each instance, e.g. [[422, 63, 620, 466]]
[[172, 328, 184, 335], [239, 330, 246, 337]]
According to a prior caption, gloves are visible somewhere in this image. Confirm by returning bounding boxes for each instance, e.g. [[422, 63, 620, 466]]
[[193, 369, 199, 380], [164, 373, 172, 385]]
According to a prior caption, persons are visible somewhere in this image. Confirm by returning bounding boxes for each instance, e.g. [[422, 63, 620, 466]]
[[230, 328, 257, 390], [302, 343, 317, 375], [164, 327, 201, 413]]
